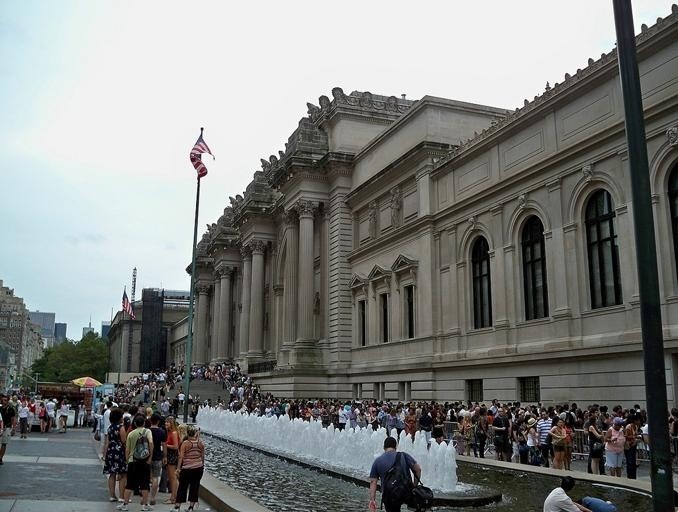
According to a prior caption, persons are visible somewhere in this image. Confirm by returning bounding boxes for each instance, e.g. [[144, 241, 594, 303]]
[[543, 476, 592, 512], [368, 437, 422, 512], [91, 359, 678, 512], [1, 392, 87, 463]]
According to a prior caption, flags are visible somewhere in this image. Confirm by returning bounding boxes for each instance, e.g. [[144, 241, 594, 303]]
[[190, 133, 215, 178], [122, 290, 136, 320]]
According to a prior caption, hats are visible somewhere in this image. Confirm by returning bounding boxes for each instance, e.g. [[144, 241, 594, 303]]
[[612, 417, 623, 424], [527, 418, 538, 428]]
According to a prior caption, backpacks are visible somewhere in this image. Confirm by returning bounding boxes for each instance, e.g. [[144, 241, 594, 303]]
[[134, 429, 151, 462], [562, 411, 574, 428], [381, 451, 409, 510]]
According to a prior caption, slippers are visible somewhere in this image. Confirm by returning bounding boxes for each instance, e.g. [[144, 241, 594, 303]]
[[162, 499, 176, 504]]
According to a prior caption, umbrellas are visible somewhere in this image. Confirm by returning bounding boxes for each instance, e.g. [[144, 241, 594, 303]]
[[70, 376, 103, 401]]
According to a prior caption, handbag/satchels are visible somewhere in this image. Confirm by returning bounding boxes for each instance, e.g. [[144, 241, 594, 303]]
[[593, 442, 605, 451], [411, 484, 434, 511], [44, 411, 48, 422]]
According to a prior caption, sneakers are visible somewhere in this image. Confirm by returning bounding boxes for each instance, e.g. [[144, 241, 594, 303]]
[[24, 435, 27, 438], [20, 435, 23, 438], [170, 506, 180, 512], [110, 497, 118, 501], [116, 503, 128, 511], [0, 459, 4, 465], [149, 500, 156, 505], [141, 499, 144, 505], [185, 508, 193, 512], [141, 504, 152, 511], [59, 431, 63, 434], [119, 498, 132, 503], [64, 431, 67, 434]]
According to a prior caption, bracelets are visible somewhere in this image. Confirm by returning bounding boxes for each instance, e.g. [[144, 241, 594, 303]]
[[370, 498, 376, 501]]
